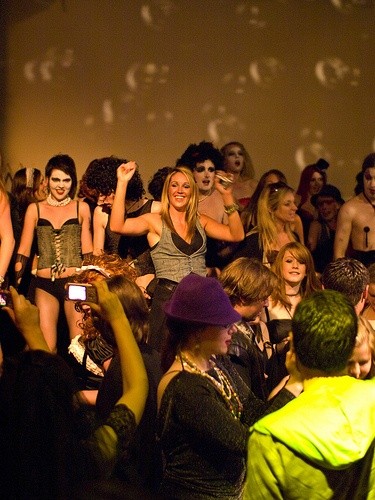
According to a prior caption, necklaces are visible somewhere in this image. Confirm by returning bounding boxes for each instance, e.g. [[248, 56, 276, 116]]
[[175, 352, 242, 420]]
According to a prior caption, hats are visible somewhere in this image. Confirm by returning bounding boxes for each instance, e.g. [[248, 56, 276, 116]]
[[162, 271, 242, 325]]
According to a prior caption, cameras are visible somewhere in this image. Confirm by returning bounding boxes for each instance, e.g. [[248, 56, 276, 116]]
[[64, 282, 98, 304], [216, 174, 232, 190], [0, 290, 14, 309]]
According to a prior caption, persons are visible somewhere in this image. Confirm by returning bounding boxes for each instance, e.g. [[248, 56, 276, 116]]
[[0, 252, 170, 500], [243, 291, 375, 500], [0, 140, 375, 353], [152, 274, 303, 500], [219, 242, 375, 402]]
